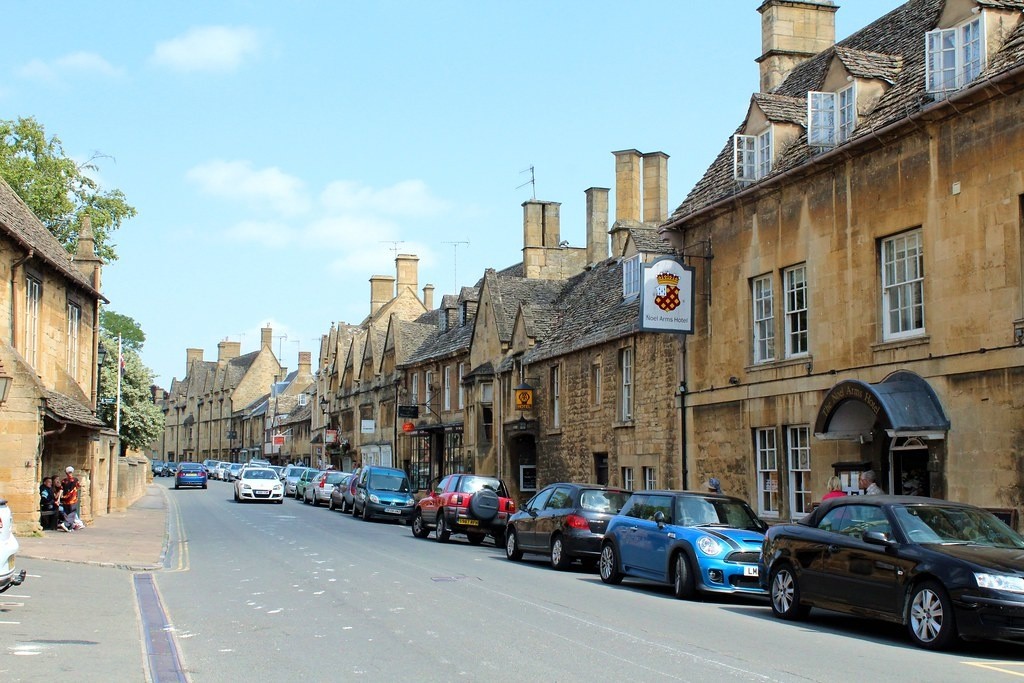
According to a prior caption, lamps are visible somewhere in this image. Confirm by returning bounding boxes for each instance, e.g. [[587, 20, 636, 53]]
[[1015, 326, 1024, 346], [806, 362, 812, 376], [678, 381, 687, 394], [319, 394, 331, 415], [728, 376, 739, 384]]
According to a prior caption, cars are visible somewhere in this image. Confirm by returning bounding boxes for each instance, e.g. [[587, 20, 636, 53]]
[[173, 462, 209, 489], [758, 495, 1023, 652], [599, 490, 770, 599], [505, 483, 635, 570], [352, 465, 419, 526], [150, 460, 359, 514], [0, 500, 26, 593], [233, 467, 285, 504]]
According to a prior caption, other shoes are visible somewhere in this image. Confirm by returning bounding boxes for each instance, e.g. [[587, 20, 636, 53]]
[[60, 523, 69, 531]]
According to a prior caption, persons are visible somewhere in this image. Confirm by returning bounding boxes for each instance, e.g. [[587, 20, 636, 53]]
[[351, 462, 362, 475], [821, 476, 846, 502], [40, 465, 85, 532], [859, 470, 885, 496], [702, 477, 721, 493]]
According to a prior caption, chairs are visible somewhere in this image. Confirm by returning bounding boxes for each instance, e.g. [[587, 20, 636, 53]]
[[833, 517, 853, 532], [39, 501, 59, 532]]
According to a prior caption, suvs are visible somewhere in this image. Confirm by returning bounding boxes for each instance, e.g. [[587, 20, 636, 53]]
[[412, 474, 515, 548]]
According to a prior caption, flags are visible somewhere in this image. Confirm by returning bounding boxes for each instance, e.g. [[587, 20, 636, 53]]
[[121, 351, 126, 372]]
